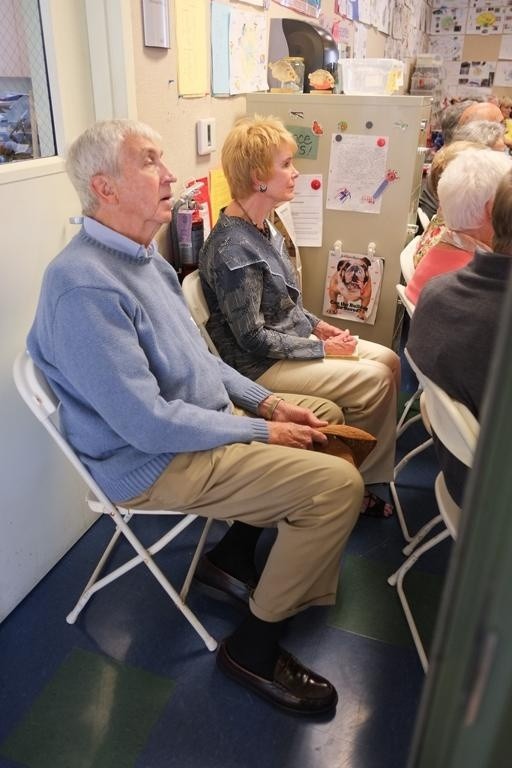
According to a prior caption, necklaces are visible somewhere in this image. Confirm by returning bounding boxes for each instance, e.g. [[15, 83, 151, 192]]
[[236, 200, 270, 240]]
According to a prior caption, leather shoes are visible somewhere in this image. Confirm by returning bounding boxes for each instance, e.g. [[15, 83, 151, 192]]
[[215, 637, 338, 715], [193, 553, 259, 607]]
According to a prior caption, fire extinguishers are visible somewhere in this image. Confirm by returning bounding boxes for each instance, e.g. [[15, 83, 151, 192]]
[[170, 182, 205, 284]]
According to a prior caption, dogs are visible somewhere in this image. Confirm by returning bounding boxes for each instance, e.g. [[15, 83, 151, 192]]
[[326, 257, 372, 321]]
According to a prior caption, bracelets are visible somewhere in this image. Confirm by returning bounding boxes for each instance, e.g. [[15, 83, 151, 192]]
[[265, 396, 284, 420]]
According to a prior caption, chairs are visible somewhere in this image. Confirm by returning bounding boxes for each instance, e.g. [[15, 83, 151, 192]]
[[390, 346, 447, 554], [392, 209, 430, 350], [387, 374, 481, 672], [13, 348, 233, 650], [182, 269, 224, 363]]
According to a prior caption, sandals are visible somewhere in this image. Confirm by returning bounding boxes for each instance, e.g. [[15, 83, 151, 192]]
[[360, 490, 394, 518]]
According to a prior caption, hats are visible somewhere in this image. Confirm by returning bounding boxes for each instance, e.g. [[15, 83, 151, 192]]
[[312, 424, 377, 469]]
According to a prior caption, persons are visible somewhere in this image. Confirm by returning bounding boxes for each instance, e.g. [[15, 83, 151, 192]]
[[197, 114, 401, 521], [407, 170, 511, 509], [418, 100, 510, 219], [25, 118, 366, 716], [405, 148, 510, 306]]
